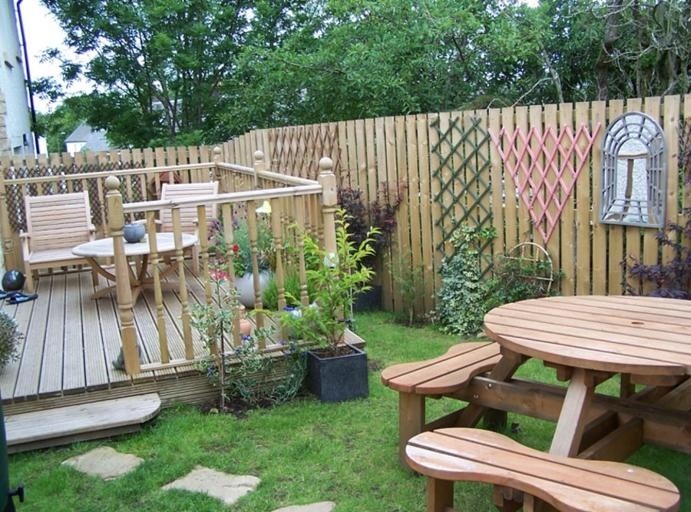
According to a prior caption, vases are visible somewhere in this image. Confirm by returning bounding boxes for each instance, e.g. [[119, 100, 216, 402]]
[[223, 270, 277, 309]]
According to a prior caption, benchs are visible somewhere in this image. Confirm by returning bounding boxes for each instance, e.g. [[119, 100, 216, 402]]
[[405, 426, 682, 511], [380, 341, 504, 475]]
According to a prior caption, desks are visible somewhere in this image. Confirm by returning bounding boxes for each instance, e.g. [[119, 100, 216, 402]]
[[439, 291, 690, 512]]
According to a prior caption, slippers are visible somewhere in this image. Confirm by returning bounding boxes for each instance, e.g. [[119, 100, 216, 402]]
[[0, 290, 38, 304]]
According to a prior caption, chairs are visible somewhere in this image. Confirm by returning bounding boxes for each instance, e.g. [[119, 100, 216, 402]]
[[17, 189, 101, 288], [130, 177, 225, 278]]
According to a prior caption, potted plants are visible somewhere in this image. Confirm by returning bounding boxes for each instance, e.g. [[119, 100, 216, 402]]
[[337, 180, 406, 312], [264, 209, 375, 406]]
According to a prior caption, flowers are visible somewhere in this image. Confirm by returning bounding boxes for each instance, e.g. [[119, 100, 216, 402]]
[[211, 215, 284, 275]]
